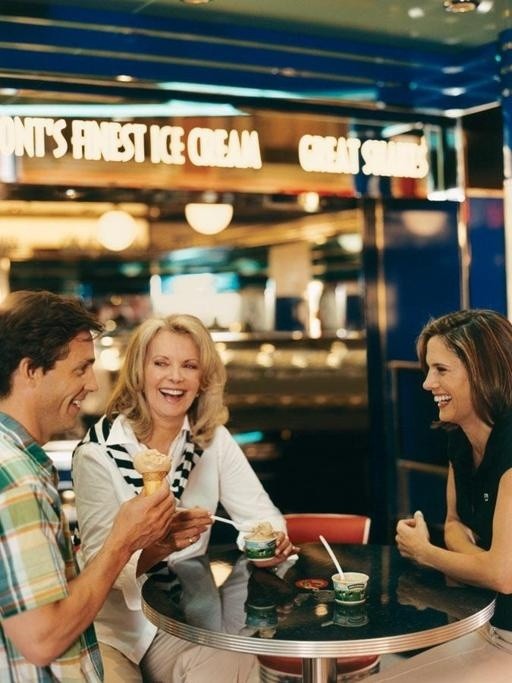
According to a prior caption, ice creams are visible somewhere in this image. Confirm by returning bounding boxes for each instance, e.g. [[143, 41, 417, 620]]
[[243, 521, 276, 541], [131, 447, 171, 497]]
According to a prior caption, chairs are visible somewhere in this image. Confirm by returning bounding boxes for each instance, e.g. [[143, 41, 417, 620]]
[[255, 514, 383, 683]]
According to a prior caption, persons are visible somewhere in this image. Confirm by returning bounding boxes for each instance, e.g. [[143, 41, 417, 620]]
[[0, 291, 177, 683], [70, 313, 302, 683], [356, 309, 512, 683]]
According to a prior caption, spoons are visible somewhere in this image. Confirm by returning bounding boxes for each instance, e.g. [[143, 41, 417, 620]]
[[176, 506, 258, 532]]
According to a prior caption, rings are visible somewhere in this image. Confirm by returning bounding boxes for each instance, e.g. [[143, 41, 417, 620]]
[[188, 538, 193, 545]]
